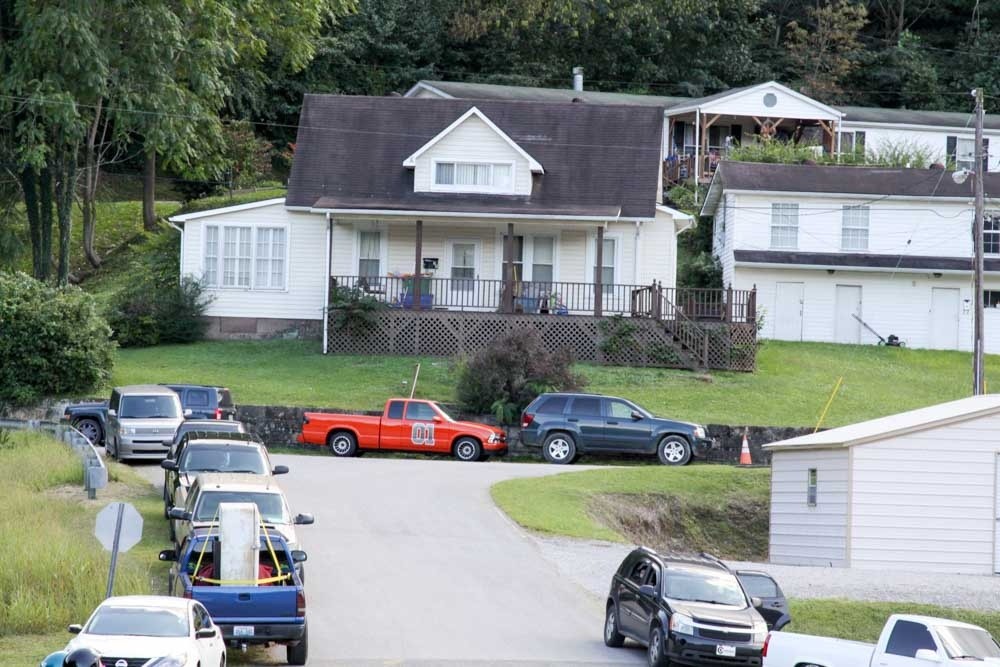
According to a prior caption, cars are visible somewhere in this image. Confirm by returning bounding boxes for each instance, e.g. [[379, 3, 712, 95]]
[[162, 418, 248, 500], [162, 438, 290, 519], [66, 593, 228, 667]]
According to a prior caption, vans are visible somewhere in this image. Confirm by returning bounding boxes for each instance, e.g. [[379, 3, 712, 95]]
[[105, 384, 193, 465]]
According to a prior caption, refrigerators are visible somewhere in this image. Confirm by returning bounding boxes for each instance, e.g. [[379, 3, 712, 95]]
[[218, 502, 260, 586]]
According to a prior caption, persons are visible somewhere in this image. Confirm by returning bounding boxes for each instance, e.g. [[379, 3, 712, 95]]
[[58, 399, 71, 425], [145, 402, 157, 418], [710, 149, 720, 177], [666, 147, 678, 180]]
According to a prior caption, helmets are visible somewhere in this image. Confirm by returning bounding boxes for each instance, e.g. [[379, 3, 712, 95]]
[[40, 651, 68, 667], [63, 649, 103, 667]]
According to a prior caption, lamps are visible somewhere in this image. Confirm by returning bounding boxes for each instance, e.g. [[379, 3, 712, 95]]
[[934, 273, 942, 278]]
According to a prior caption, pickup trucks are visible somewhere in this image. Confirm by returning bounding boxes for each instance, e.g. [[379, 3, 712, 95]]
[[296, 398, 509, 462], [761, 612, 1000, 667], [158, 527, 308, 666], [167, 472, 314, 552]]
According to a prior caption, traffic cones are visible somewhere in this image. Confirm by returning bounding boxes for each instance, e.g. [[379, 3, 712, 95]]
[[736, 434, 755, 466]]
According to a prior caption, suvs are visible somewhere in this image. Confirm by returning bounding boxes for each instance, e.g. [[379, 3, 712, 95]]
[[519, 392, 708, 465], [63, 383, 237, 448], [603, 545, 791, 667]]
